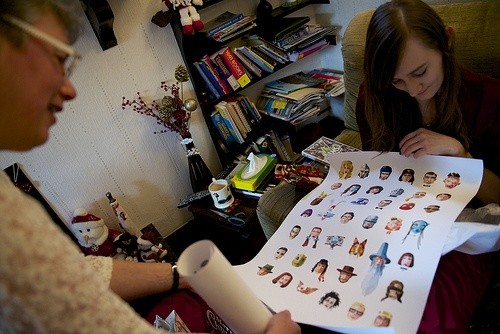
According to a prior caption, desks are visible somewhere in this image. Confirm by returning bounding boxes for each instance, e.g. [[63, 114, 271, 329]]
[[188, 191, 267, 266]]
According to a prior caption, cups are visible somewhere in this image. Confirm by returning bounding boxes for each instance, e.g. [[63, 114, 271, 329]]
[[208, 178, 234, 209]]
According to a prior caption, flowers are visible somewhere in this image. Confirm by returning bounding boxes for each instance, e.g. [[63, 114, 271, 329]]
[[122, 65, 198, 139]]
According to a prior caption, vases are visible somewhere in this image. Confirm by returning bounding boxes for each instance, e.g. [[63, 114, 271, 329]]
[[183, 139, 214, 194]]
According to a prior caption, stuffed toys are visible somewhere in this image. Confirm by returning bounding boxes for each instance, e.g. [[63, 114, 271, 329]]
[[71, 208, 168, 263], [162, 0, 204, 35]]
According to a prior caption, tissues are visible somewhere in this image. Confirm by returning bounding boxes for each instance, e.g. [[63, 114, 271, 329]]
[[231, 153, 278, 190]]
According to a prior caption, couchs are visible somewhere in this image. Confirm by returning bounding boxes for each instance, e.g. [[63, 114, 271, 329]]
[[257, 1, 500, 240]]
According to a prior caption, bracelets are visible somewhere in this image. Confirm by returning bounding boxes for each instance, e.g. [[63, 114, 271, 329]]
[[170, 262, 180, 291]]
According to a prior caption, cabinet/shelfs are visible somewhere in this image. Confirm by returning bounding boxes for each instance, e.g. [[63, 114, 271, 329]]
[[151, 0, 345, 168]]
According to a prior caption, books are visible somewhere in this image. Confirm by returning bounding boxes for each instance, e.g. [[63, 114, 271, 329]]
[[302, 136, 361, 165], [200, 11, 257, 42], [245, 130, 293, 161], [211, 69, 346, 147], [193, 16, 337, 98]]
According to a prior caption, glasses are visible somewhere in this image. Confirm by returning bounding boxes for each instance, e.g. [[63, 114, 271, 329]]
[[0, 16, 82, 80]]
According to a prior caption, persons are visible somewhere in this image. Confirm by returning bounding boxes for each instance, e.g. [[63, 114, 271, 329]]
[[0, 0, 302, 334], [355, 0, 500, 334]]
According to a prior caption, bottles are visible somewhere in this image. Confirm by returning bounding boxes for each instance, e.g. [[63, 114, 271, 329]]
[[105, 192, 143, 238]]
